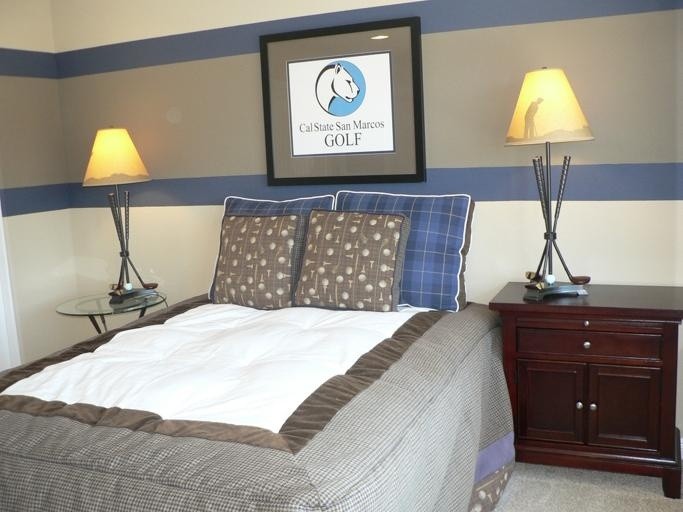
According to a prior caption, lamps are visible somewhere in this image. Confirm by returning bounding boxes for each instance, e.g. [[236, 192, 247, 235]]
[[79, 126, 159, 298], [503, 65, 597, 305]]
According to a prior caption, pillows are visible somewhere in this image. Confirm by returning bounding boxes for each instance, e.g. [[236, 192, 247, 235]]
[[208, 206, 415, 313]]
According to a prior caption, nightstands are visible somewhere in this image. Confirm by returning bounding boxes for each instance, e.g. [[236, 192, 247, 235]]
[[488, 282, 683, 500]]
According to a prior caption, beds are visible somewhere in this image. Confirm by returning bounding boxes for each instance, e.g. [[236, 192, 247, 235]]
[[0, 291, 503, 512]]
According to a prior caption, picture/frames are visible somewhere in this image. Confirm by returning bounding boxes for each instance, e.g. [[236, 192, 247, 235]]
[[257, 15, 428, 188]]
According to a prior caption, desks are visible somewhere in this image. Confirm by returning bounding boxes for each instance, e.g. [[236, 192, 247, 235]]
[[55, 294, 167, 336]]
